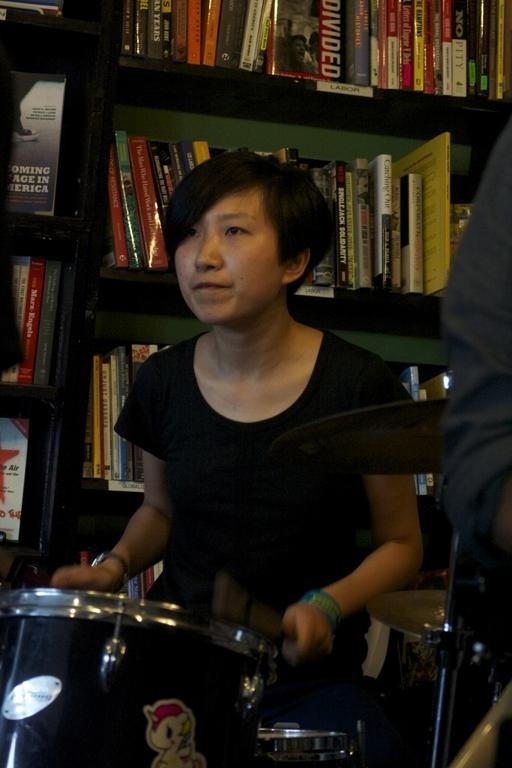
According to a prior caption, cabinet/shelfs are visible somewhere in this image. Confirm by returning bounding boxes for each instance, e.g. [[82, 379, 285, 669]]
[[0, 1, 511, 589]]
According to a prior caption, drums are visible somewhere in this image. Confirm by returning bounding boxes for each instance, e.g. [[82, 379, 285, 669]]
[[0, 587, 278, 766], [259, 729, 355, 766]]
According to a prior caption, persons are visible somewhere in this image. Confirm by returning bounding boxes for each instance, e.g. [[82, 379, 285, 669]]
[[50, 147, 439, 767], [427, 117, 512, 766], [287, 34, 307, 72]]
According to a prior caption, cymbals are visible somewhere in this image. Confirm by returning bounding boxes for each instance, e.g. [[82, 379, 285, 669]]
[[367, 591, 452, 644], [270, 398, 447, 473]]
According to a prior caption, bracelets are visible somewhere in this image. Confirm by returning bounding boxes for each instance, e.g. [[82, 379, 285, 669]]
[[302, 586, 344, 635]]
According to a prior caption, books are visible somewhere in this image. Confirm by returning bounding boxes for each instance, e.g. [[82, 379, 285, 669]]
[[396, 356, 461, 506], [0, 1, 63, 17], [122, 1, 510, 101], [4, 71, 66, 216], [0, 249, 166, 603], [106, 132, 473, 296]]
